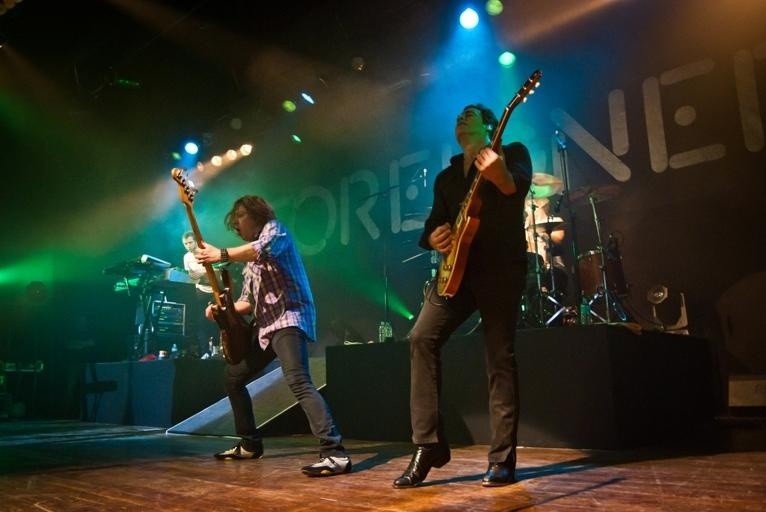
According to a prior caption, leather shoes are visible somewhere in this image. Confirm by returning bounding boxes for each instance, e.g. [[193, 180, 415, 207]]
[[482, 462, 515, 487], [393, 434, 451, 487]]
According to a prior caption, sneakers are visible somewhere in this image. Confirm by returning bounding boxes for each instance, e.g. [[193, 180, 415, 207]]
[[301, 455, 352, 476], [214, 441, 263, 459]]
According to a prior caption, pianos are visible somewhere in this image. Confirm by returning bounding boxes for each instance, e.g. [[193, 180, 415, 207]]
[[107, 266, 195, 292]]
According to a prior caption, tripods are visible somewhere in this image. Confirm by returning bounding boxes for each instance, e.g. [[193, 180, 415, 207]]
[[588, 196, 630, 323], [522, 213, 581, 327], [543, 145, 608, 327]]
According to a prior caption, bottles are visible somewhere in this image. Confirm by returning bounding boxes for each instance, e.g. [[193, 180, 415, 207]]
[[378, 321, 385, 343], [384, 321, 392, 337], [580, 291, 587, 303], [208, 337, 215, 351], [171, 344, 178, 352], [520, 295, 527, 319]]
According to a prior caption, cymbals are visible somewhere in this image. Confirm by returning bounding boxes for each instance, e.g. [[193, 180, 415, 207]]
[[565, 180, 623, 206], [526, 215, 568, 236], [524, 172, 563, 199]]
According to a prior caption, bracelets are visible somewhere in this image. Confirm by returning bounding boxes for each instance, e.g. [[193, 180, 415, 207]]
[[219, 247, 228, 262]]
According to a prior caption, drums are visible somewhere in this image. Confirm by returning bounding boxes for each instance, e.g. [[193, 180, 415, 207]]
[[576, 246, 630, 303], [519, 251, 547, 324]]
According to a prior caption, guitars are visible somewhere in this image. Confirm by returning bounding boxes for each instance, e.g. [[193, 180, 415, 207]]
[[170, 166, 252, 365], [435, 67, 543, 299]]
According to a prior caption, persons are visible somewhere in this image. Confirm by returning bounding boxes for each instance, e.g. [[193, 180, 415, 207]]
[[194, 195, 352, 477], [180, 231, 227, 362], [392, 103, 533, 487], [521, 194, 566, 267]]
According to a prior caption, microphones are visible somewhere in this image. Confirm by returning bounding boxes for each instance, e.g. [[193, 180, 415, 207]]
[[551, 129, 569, 155], [420, 167, 432, 189], [552, 189, 567, 213]]
